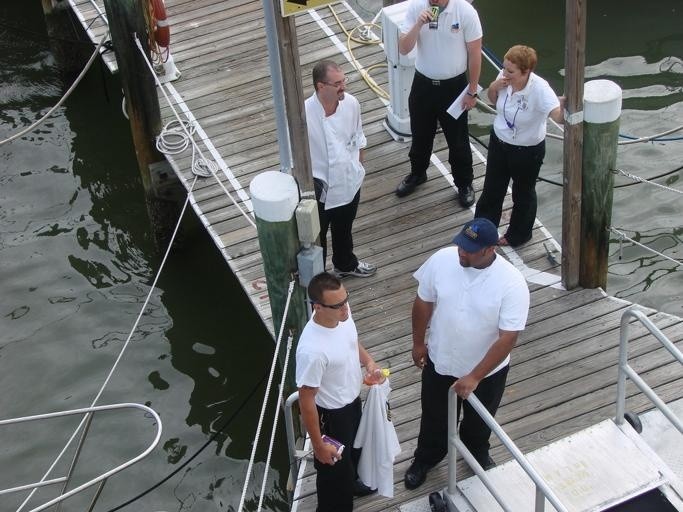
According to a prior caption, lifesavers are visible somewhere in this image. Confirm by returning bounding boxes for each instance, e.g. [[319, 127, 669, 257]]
[[150, 1, 170, 48]]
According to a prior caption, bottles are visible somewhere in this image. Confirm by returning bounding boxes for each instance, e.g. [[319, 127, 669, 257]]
[[362, 368, 391, 385], [428, 2, 439, 29]]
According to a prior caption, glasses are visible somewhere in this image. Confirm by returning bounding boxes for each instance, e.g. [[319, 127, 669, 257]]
[[309, 290, 349, 309], [318, 75, 349, 88]]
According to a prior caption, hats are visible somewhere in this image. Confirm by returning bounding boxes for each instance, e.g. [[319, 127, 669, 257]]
[[451, 217, 498, 254]]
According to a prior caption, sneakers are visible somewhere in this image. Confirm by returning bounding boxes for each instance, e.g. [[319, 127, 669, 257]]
[[333, 260, 377, 278]]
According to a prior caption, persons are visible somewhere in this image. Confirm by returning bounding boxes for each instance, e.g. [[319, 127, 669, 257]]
[[402, 217, 531, 490], [293, 270, 387, 511], [395, 0, 483, 208], [301, 59, 377, 279], [471, 45, 568, 247]]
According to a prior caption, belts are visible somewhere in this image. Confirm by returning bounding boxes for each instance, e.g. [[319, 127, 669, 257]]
[[415, 68, 467, 87]]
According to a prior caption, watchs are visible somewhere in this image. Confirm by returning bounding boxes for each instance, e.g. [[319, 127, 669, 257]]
[[465, 90, 478, 99]]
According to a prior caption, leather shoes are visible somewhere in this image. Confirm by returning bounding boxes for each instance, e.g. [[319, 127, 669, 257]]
[[497, 236, 509, 246], [481, 456, 497, 471], [396, 171, 427, 196], [405, 459, 435, 490], [354, 478, 378, 495], [459, 183, 474, 207]]
